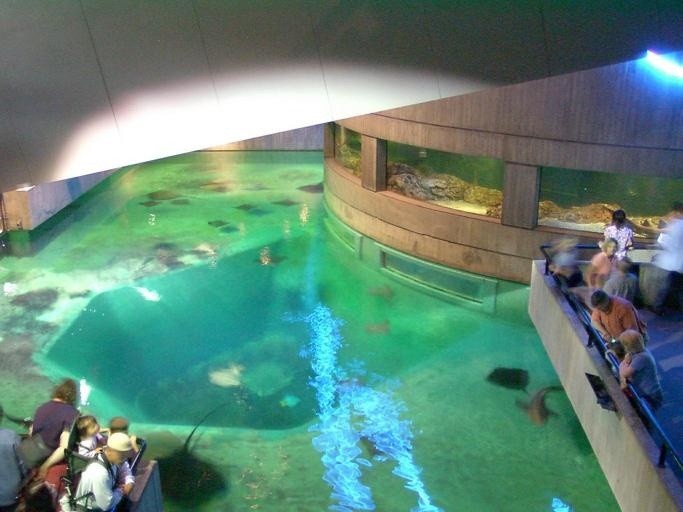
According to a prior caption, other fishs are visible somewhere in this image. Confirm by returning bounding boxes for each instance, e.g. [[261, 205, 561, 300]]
[[516, 386, 564, 425], [487, 368, 529, 397], [155, 401, 233, 504]]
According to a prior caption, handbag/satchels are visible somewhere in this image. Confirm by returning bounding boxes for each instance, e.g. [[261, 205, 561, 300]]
[[634, 318, 650, 345]]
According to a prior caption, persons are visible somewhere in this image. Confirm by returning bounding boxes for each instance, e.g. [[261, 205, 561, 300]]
[[0, 378, 141, 512], [586, 201, 683, 414]]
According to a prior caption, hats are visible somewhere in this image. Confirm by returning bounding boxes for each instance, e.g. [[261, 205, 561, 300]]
[[107, 433, 132, 451]]
